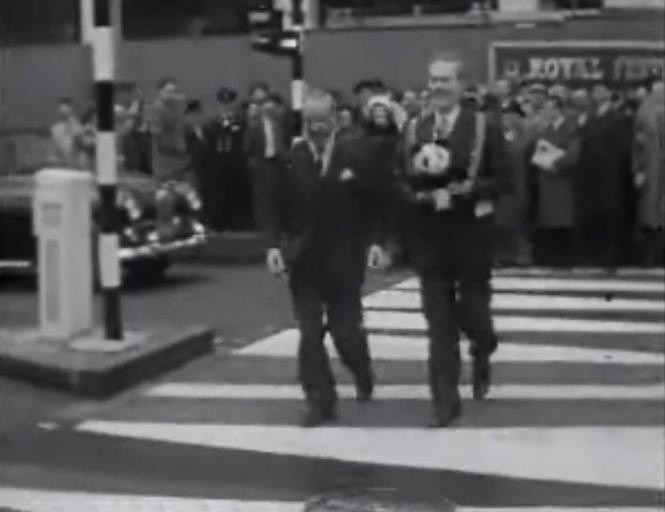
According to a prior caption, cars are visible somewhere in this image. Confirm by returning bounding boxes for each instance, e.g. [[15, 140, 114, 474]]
[[0, 126, 208, 280]]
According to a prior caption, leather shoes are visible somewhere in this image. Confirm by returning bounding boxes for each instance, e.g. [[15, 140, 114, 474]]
[[303, 400, 339, 424], [430, 403, 461, 427], [354, 369, 373, 401], [473, 361, 492, 402]]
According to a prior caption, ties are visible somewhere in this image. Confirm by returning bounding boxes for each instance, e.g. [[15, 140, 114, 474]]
[[314, 144, 324, 172], [435, 118, 448, 140]]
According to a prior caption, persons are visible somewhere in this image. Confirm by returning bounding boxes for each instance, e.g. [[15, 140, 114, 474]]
[[398, 58, 497, 430], [261, 89, 394, 427]]
